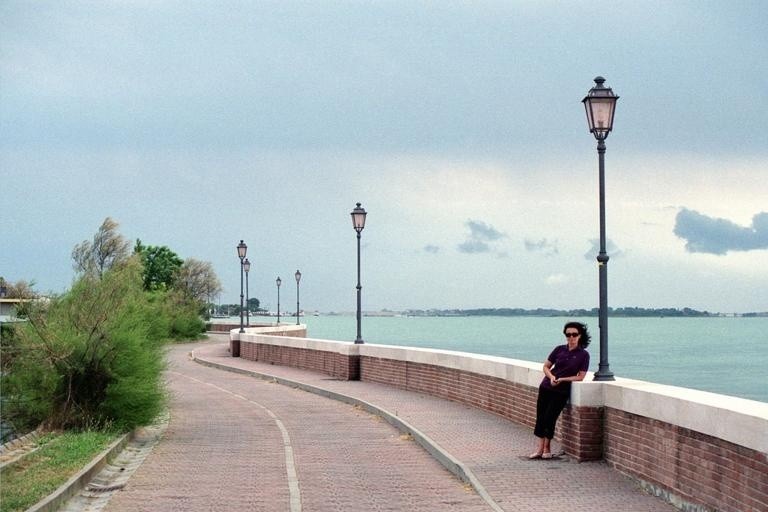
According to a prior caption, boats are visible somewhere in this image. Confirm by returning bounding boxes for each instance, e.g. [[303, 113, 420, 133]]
[[313, 311, 319, 316], [269, 309, 304, 317], [248, 310, 252, 317]]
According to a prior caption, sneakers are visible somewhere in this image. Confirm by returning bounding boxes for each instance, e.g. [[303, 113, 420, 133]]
[[542, 452, 552, 459], [527, 452, 542, 459]]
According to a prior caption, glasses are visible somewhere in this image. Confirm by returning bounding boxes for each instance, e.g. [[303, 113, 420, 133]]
[[565, 332, 580, 337]]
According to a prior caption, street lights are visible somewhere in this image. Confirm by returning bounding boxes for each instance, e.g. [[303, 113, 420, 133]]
[[242, 258, 250, 328], [352, 201, 368, 345], [235, 238, 252, 332], [272, 275, 283, 325], [295, 269, 303, 324], [582, 75, 620, 381]]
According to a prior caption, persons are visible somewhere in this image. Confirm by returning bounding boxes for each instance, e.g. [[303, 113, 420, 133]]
[[530, 323, 591, 458]]
